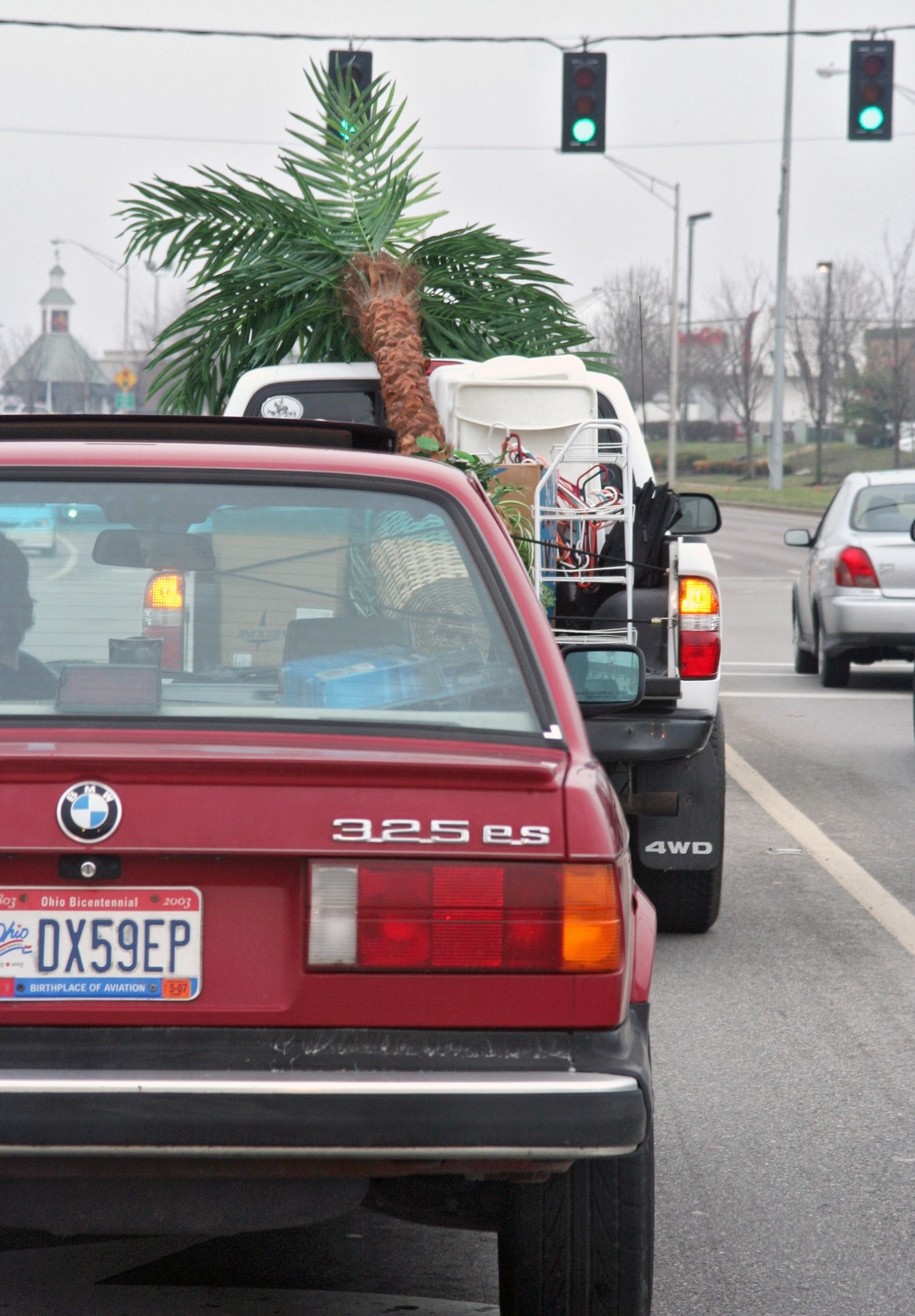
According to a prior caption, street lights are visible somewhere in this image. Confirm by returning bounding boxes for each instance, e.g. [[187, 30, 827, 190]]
[[143, 258, 170, 357], [811, 259, 836, 483], [47, 236, 130, 351], [680, 204, 715, 452]]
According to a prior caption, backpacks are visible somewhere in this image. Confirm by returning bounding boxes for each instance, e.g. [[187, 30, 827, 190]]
[[599, 477, 682, 590]]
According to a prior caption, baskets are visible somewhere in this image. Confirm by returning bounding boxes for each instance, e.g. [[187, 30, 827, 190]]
[[348, 507, 491, 665]]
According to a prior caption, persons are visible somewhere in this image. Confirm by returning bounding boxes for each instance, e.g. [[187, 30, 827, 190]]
[[135, 533, 184, 569], [0, 533, 59, 701]]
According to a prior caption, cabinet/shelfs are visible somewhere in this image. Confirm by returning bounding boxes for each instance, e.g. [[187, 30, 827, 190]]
[[530, 418, 635, 645]]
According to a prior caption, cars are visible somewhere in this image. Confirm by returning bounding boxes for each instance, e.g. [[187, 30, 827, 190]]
[[0, 404, 663, 1316], [0, 503, 58, 560], [188, 503, 242, 534], [781, 470, 915, 691], [49, 501, 106, 531]]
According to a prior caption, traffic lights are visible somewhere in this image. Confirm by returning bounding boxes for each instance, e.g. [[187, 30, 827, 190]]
[[323, 47, 378, 157], [119, 370, 128, 392], [555, 46, 611, 156], [846, 37, 895, 141]]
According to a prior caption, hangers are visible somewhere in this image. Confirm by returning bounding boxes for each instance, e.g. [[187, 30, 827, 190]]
[[500, 429, 624, 592]]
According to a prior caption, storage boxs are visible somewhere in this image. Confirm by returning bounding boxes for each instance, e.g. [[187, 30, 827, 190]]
[[491, 462, 558, 633]]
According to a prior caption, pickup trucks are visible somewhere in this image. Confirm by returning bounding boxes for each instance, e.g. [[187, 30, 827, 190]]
[[93, 351, 725, 934]]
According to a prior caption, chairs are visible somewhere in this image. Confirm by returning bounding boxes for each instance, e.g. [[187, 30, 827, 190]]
[[283, 614, 409, 655]]
